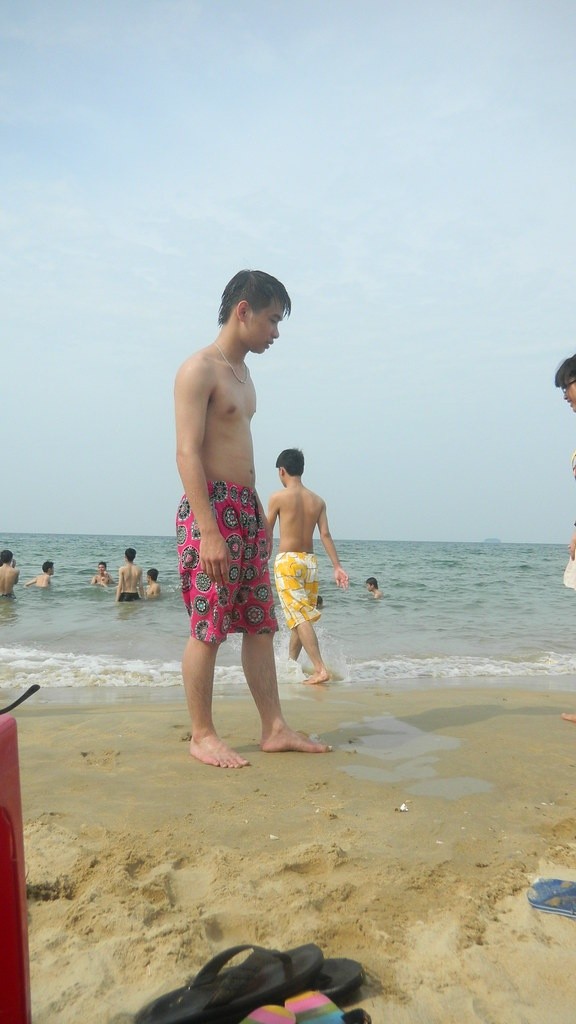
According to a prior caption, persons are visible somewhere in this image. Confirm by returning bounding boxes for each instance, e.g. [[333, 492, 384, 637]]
[[24, 560, 54, 587], [116, 547, 144, 602], [551, 352, 576, 722], [365, 577, 382, 598], [266, 447, 349, 685], [0, 549, 19, 598], [89, 563, 160, 599], [175, 270, 334, 768]]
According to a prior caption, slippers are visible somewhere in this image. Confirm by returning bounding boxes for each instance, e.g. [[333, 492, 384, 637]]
[[528, 877, 576, 920], [133, 942, 372, 1024]]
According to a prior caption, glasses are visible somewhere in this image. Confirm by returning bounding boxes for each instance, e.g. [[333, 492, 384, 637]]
[[561, 380, 576, 394]]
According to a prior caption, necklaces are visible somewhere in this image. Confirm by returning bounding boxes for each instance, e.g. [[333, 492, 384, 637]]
[[213, 341, 248, 384]]
